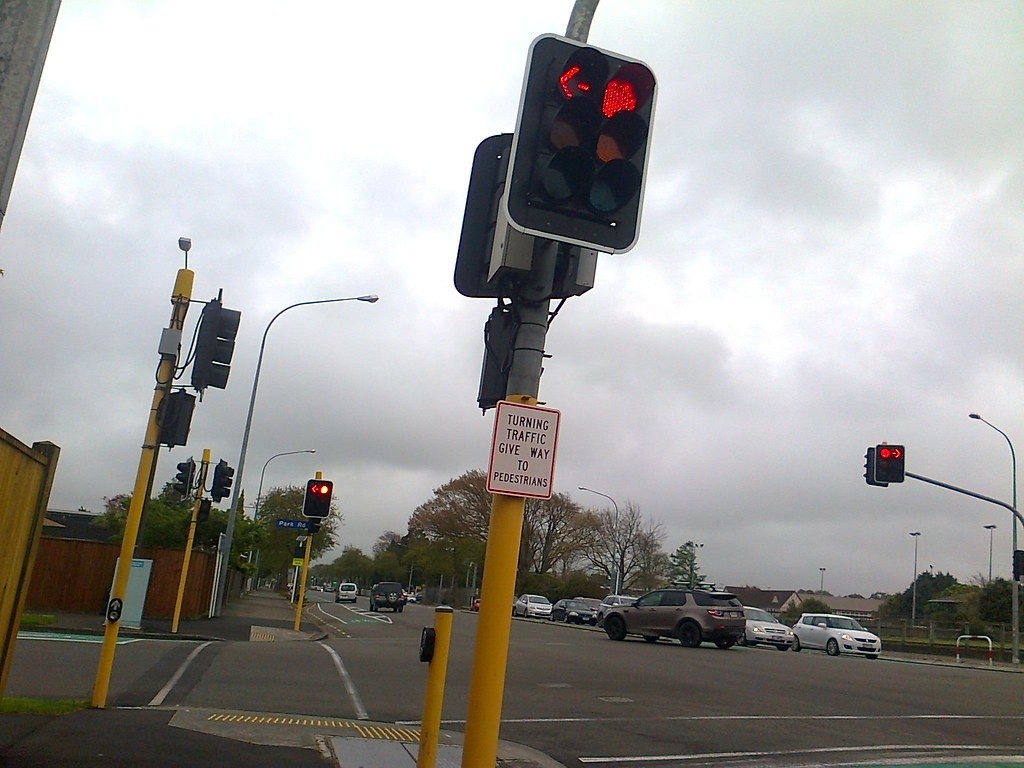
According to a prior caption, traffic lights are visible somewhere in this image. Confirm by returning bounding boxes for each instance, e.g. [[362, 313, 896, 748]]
[[302, 479, 333, 517], [863, 447, 888, 487], [174, 456, 194, 501], [211, 459, 234, 502], [191, 300, 242, 389], [876, 445, 905, 483], [505, 35, 657, 254]]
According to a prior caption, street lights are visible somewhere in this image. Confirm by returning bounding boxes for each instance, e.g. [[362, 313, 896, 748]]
[[983, 524, 997, 582], [577, 486, 619, 593], [212, 294, 380, 618], [254, 448, 316, 521], [396, 543, 415, 592], [686, 542, 704, 590], [909, 532, 921, 619], [969, 412, 1021, 664], [819, 567, 826, 591]]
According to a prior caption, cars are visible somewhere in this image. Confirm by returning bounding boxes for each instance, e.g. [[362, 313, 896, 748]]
[[515, 594, 602, 626], [474, 598, 480, 611], [401, 588, 423, 603], [791, 612, 882, 659], [308, 583, 334, 592], [734, 606, 794, 651]]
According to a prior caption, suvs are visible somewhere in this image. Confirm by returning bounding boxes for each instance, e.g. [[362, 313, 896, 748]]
[[604, 582, 746, 648], [597, 596, 639, 627], [370, 582, 407, 612]]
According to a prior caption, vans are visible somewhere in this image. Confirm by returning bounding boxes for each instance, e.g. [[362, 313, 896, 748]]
[[335, 583, 358, 603]]
